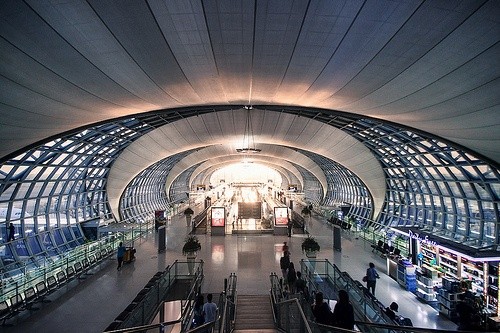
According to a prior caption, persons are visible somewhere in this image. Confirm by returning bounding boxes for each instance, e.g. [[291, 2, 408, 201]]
[[366, 262, 379, 297], [390, 302, 398, 319], [454, 294, 486, 333], [280, 219, 354, 333], [183, 207, 195, 226], [7, 223, 15, 242], [193, 294, 218, 333], [117, 242, 126, 271]]
